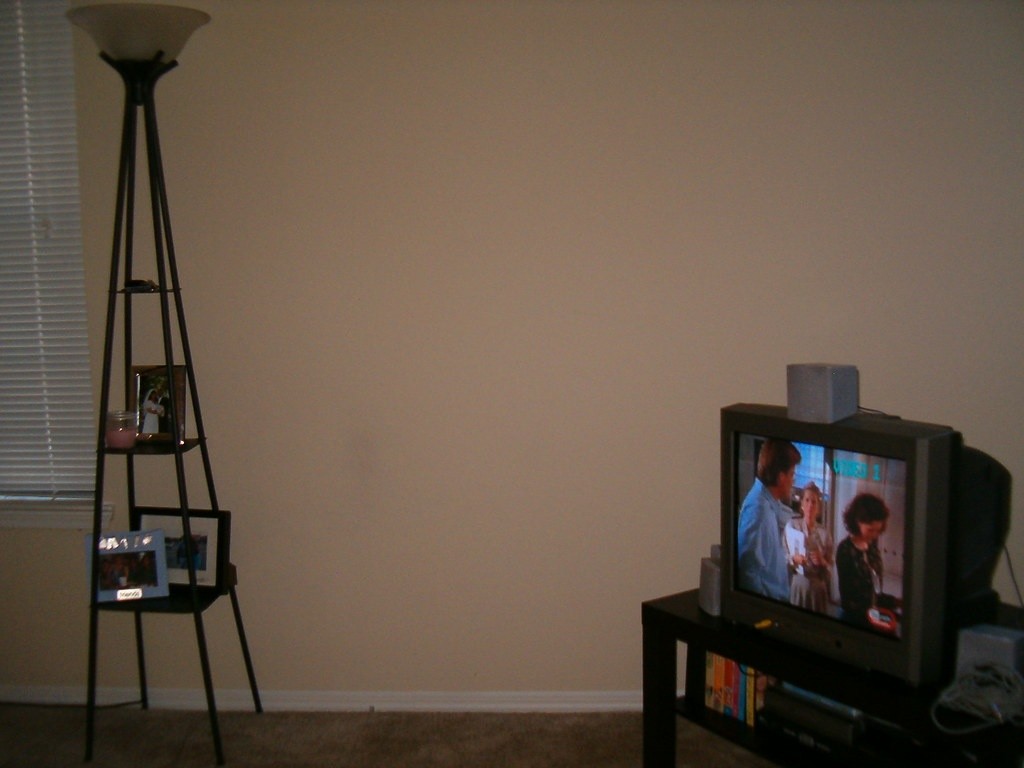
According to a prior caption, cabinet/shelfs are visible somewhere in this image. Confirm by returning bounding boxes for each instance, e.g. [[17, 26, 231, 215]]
[[641, 588, 1024, 768]]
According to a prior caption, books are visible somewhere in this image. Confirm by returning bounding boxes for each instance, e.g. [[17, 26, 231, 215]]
[[703, 651, 781, 727]]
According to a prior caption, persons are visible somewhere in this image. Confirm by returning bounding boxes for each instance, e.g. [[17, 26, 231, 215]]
[[736, 438, 802, 603], [781, 482, 834, 614], [836, 492, 891, 619], [142, 387, 169, 435], [99, 552, 157, 590]]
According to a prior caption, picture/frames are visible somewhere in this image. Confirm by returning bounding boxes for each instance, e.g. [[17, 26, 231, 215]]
[[84, 528, 169, 604], [129, 362, 187, 443], [128, 504, 231, 596]]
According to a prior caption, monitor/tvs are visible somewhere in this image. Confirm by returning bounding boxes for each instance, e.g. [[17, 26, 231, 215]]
[[718, 402, 1012, 694]]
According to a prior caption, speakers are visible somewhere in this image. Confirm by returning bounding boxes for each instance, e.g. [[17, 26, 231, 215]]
[[697, 557, 722, 617], [956, 625, 1024, 683], [787, 361, 859, 424]]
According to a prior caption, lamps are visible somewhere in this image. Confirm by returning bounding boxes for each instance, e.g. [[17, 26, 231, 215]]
[[63, 5, 262, 768]]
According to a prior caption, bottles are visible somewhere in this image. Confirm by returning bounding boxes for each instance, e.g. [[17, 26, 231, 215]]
[[106, 410, 138, 449]]
